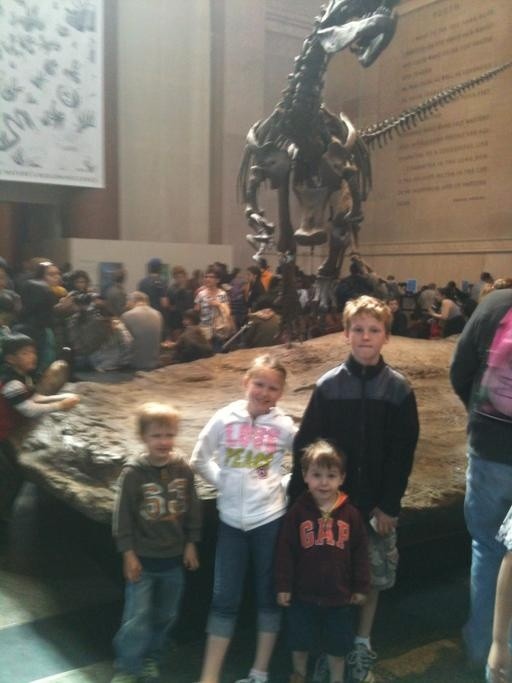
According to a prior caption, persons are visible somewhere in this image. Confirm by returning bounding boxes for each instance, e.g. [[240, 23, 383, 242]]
[[387, 298, 408, 336], [229, 267, 246, 315], [450, 287, 512, 667], [137, 257, 176, 313], [87, 303, 134, 372], [426, 287, 466, 338], [336, 262, 368, 321], [22, 262, 74, 375], [120, 290, 163, 370], [189, 353, 298, 683], [0, 289, 13, 355], [416, 282, 436, 319], [377, 274, 398, 296], [194, 269, 228, 326], [241, 293, 279, 347], [479, 273, 493, 297], [0, 257, 8, 291], [257, 257, 271, 292], [194, 272, 206, 298], [271, 439, 370, 683], [102, 267, 128, 317], [187, 269, 202, 288], [163, 266, 187, 329], [285, 294, 419, 683], [276, 255, 286, 274], [67, 269, 91, 313], [0, 333, 80, 510], [486, 503, 512, 680], [111, 401, 205, 683], [161, 308, 211, 360], [243, 266, 262, 306]]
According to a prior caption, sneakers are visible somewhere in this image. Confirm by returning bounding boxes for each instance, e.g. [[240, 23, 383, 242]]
[[141, 656, 160, 681], [111, 668, 138, 683], [346, 643, 377, 682], [312, 653, 330, 683]]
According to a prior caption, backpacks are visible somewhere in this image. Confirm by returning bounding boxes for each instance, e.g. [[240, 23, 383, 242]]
[[472, 305, 511, 421]]
